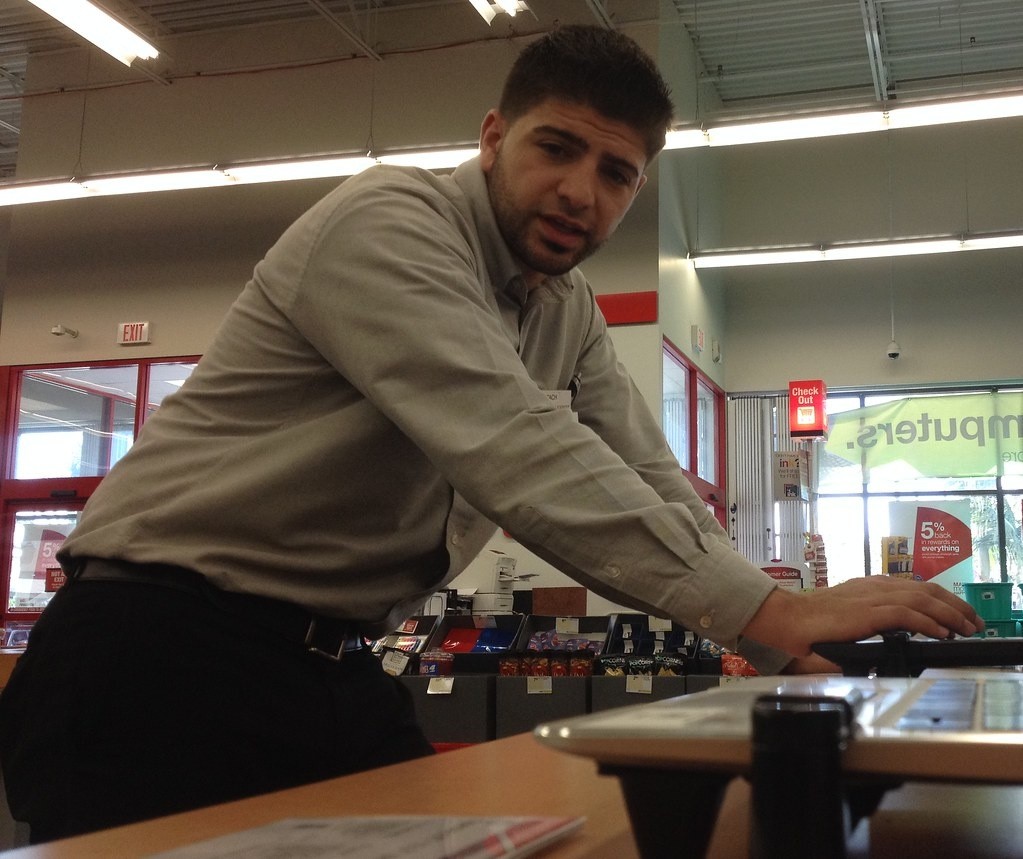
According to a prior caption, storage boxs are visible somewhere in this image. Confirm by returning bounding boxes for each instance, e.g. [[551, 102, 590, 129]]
[[962, 582, 1023, 637], [363, 550, 740, 673]]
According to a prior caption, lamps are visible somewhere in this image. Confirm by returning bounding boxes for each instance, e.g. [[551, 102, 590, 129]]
[[0, 89, 1023, 206], [886, 159, 901, 362], [689, 228, 1023, 270], [27, 0, 161, 66], [469, 0, 531, 28]]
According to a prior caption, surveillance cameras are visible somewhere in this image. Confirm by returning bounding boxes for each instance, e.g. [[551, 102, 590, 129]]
[[885, 341, 901, 361]]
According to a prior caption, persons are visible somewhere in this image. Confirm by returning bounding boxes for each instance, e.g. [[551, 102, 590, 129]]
[[0, 19, 988, 859]]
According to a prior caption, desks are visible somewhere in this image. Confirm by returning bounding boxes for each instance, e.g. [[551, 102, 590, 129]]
[[531, 674, 1023, 857], [1, 729, 1023, 859]]
